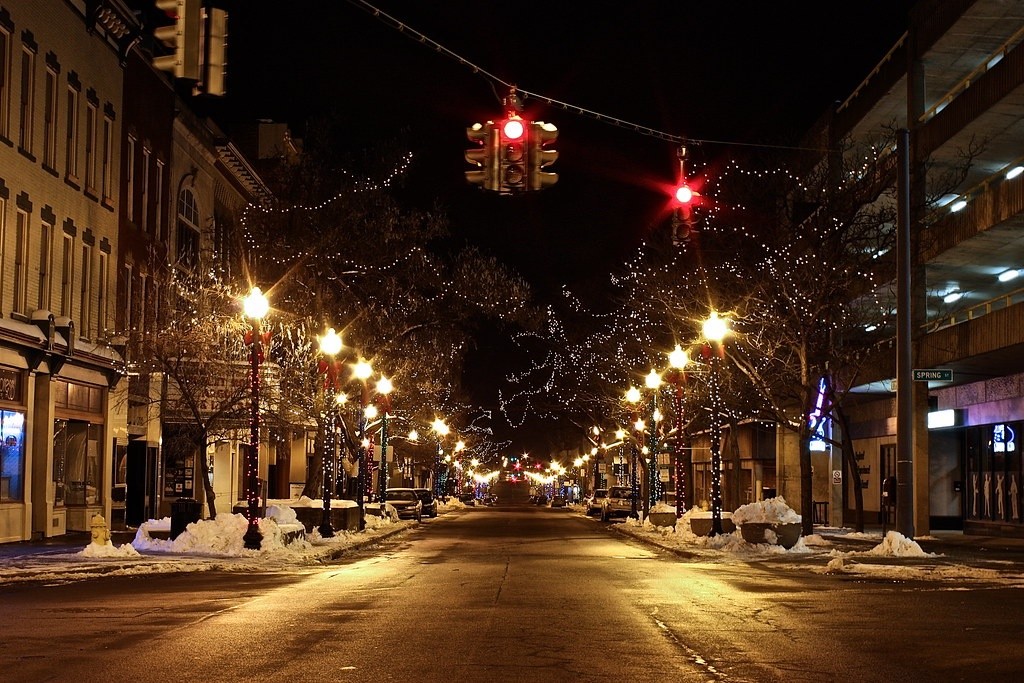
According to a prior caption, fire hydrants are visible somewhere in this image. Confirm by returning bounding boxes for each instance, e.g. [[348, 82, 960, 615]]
[[90, 513, 112, 546]]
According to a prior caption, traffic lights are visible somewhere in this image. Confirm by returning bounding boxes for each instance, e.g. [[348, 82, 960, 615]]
[[152, 0, 227, 98], [672, 187, 693, 242], [534, 121, 559, 191], [499, 119, 529, 192], [465, 124, 491, 188]]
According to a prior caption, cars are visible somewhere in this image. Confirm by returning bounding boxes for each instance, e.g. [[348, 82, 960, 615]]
[[551, 496, 565, 507], [587, 489, 610, 515], [649, 492, 676, 508], [413, 489, 438, 518], [385, 487, 422, 523]]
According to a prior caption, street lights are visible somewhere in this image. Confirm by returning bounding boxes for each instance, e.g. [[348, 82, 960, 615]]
[[376, 376, 389, 518], [353, 361, 370, 528], [322, 327, 343, 537], [242, 285, 267, 550], [669, 345, 687, 514], [702, 311, 726, 537], [626, 386, 641, 519], [646, 370, 660, 505]]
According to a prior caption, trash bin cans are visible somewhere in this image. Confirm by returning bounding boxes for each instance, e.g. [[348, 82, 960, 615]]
[[169, 497, 204, 541]]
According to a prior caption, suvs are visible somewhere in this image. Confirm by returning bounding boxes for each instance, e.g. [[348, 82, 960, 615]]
[[602, 486, 640, 522]]
